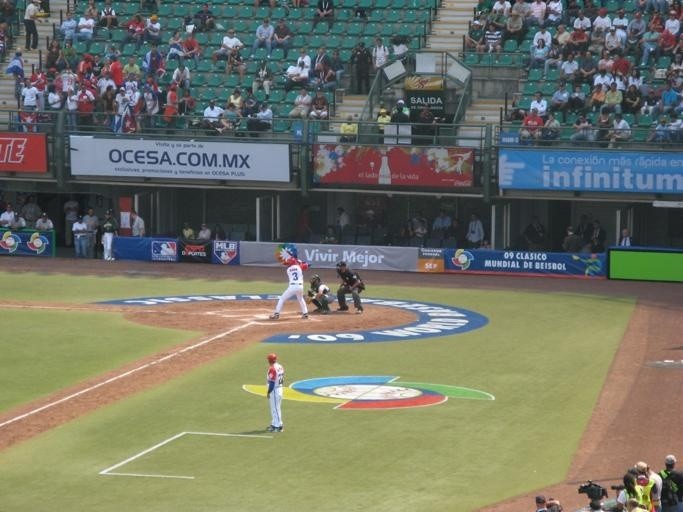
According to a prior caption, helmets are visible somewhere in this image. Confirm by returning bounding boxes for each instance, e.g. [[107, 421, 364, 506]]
[[336, 261, 346, 273], [310, 274, 320, 288], [290, 258, 296, 265], [267, 353, 277, 362]]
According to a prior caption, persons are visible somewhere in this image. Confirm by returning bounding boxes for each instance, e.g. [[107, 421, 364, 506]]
[[0, 195, 54, 231], [306, 274, 332, 314], [336, 260, 364, 314], [269, 258, 309, 318], [297, 206, 350, 245], [532, 455, 682, 511], [266, 353, 284, 431], [63, 194, 145, 259], [523, 216, 634, 254], [405, 208, 492, 250], [0, 0, 683, 149], [181, 221, 226, 241]]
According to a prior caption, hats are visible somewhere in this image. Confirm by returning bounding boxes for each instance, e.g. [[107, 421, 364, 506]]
[[664, 455, 677, 466], [635, 461, 647, 473]]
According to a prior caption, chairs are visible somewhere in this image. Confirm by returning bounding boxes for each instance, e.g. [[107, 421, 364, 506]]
[[0, 0, 683, 142]]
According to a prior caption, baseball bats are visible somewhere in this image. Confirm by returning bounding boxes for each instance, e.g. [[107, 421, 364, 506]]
[[286, 250, 304, 264]]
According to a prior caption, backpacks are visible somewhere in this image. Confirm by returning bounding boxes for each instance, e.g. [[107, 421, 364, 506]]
[[660, 470, 679, 505]]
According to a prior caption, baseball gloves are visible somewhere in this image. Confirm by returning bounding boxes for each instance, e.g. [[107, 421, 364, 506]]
[[304, 295, 312, 304]]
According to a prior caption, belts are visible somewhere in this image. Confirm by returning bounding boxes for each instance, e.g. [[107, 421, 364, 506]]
[[290, 283, 303, 285]]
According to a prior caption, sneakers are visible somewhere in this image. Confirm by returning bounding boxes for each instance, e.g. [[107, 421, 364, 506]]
[[267, 426, 282, 432], [302, 314, 309, 319], [337, 307, 348, 312], [269, 313, 279, 319], [355, 308, 363, 314]]
